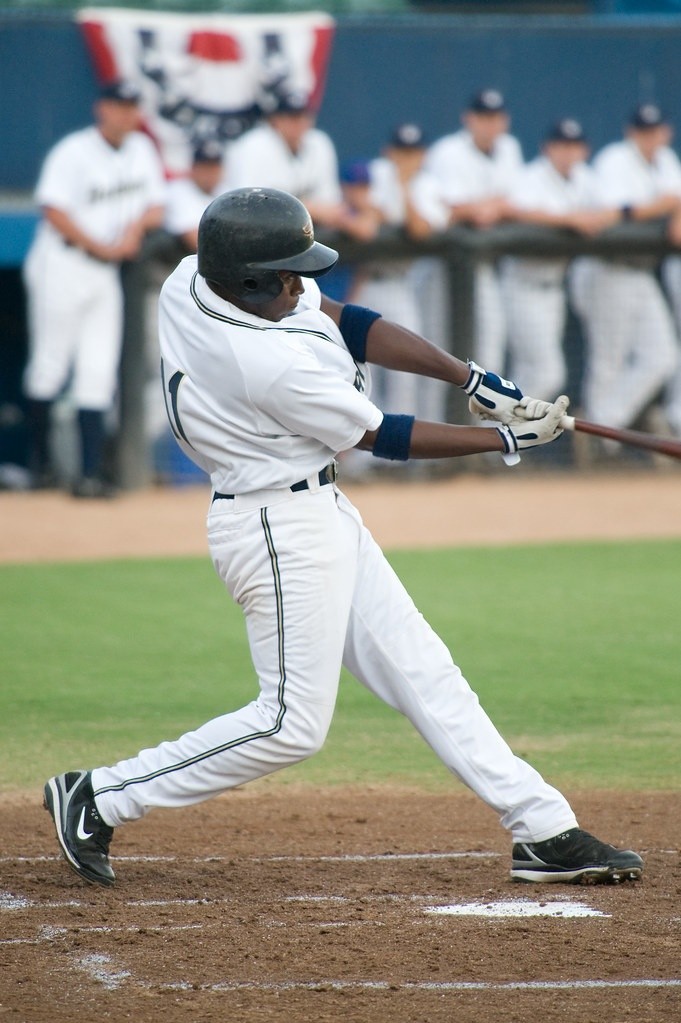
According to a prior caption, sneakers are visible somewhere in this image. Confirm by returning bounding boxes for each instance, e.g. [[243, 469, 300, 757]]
[[510, 824, 645, 886], [43, 769, 118, 890]]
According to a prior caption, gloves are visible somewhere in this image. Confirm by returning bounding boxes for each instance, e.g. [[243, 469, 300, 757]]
[[493, 398, 573, 466], [459, 360, 525, 425]]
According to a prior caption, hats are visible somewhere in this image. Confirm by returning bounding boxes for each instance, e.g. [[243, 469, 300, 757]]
[[393, 123, 427, 150], [633, 105, 666, 130], [259, 80, 309, 115], [551, 118, 584, 143], [102, 80, 142, 103], [471, 88, 504, 116]]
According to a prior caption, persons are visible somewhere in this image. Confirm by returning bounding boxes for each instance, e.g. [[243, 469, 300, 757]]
[[20, 69, 681, 500], [42, 188, 644, 889]]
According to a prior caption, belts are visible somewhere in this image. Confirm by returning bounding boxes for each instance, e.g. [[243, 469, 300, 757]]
[[213, 461, 338, 502]]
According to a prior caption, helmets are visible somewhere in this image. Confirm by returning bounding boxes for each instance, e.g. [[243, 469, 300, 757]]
[[194, 185, 338, 299]]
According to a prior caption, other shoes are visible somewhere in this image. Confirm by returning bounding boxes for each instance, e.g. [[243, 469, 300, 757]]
[[71, 477, 117, 499]]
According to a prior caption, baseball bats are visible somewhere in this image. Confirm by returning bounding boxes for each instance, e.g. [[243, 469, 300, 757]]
[[469, 396, 681, 462]]
